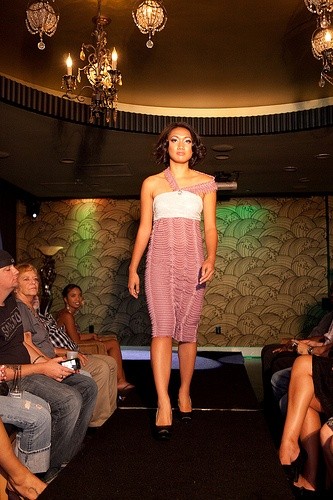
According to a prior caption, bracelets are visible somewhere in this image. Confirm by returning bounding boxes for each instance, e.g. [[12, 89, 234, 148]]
[[0, 365, 7, 381], [32, 356, 42, 363]]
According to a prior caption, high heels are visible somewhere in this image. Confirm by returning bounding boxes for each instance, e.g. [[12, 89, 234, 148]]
[[283, 454, 301, 489]]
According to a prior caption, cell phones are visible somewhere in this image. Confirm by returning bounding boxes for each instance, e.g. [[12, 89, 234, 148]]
[[59, 357, 82, 371]]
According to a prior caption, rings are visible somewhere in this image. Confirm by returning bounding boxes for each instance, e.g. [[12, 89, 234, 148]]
[[61, 375, 64, 379]]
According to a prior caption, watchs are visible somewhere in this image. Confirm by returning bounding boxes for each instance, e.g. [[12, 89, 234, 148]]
[[307, 347, 314, 355]]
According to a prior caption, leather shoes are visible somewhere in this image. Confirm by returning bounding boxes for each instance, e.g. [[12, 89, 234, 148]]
[[179, 403, 192, 426], [152, 408, 172, 440]]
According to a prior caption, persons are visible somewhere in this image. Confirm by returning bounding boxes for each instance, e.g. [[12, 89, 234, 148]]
[[127, 122, 218, 441], [0, 416, 64, 500], [12, 263, 93, 379], [0, 250, 98, 472], [56, 284, 135, 400], [33, 296, 118, 434], [0, 370, 50, 483], [279, 347, 333, 496], [261, 312, 333, 421]]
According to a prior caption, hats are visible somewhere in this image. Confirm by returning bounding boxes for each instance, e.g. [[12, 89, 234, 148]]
[[0, 249, 14, 268]]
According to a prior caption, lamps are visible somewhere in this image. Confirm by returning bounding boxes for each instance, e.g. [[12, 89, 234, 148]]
[[131, 0, 168, 48], [304, 0, 333, 87], [25, 0, 59, 51], [61, 0, 123, 128]]
